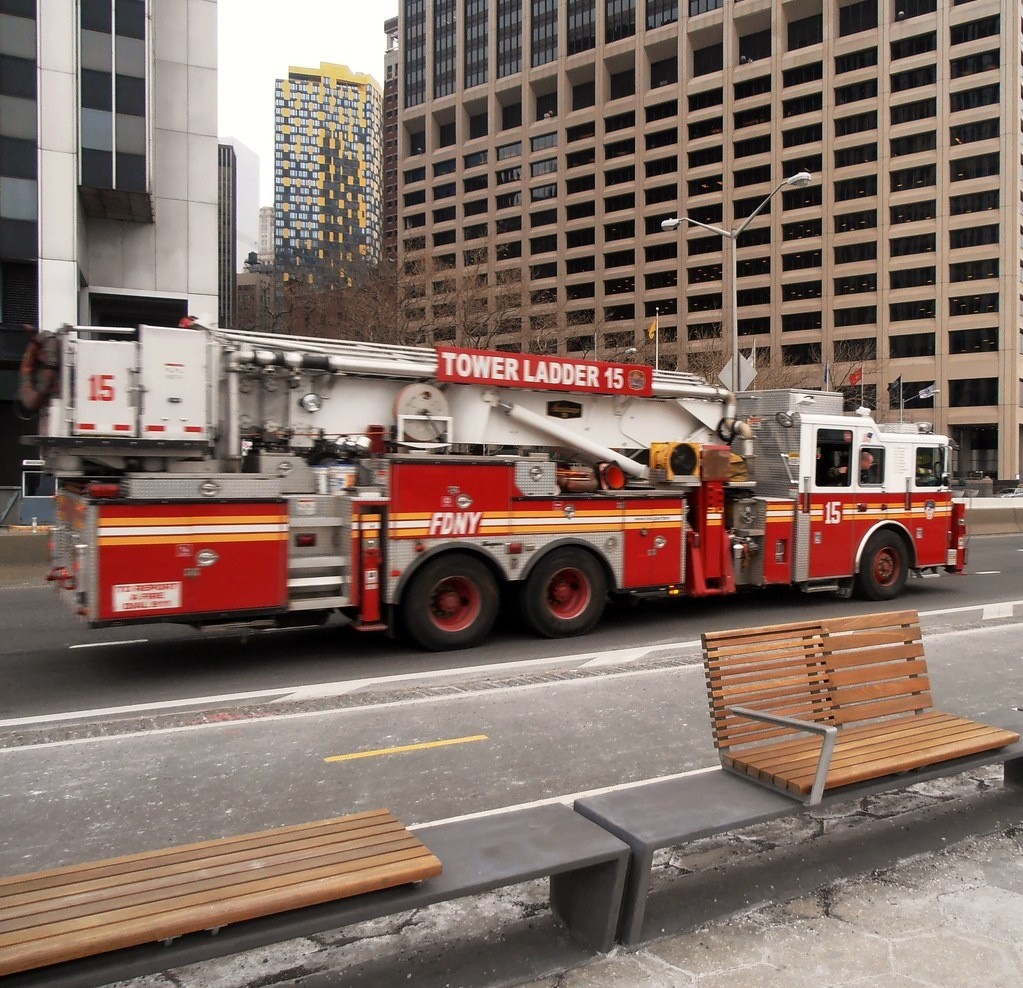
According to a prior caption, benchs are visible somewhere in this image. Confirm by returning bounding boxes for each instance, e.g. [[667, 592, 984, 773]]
[[1, 611, 1023, 987]]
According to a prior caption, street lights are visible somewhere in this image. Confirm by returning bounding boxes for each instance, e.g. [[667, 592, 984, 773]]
[[659, 170, 812, 392]]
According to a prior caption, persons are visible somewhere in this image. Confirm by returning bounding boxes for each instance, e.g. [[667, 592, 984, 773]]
[[830, 452, 873, 486]]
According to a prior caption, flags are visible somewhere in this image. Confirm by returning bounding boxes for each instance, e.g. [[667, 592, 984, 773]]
[[849, 368, 862, 385], [648, 318, 656, 339], [887, 377, 900, 392], [824, 361, 828, 383], [919, 385, 934, 398]]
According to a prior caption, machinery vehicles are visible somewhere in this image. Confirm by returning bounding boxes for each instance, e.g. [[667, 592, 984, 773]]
[[16, 317, 969, 651]]
